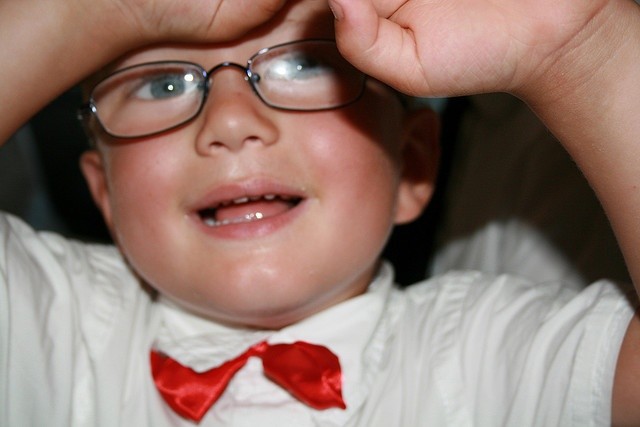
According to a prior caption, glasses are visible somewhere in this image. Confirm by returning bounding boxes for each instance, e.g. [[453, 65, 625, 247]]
[[74, 32, 368, 142]]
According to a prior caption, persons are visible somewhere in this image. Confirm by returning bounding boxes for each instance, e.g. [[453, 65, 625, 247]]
[[0, 0, 640, 427]]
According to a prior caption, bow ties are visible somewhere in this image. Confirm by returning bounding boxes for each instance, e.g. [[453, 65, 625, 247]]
[[148, 340, 348, 424]]
